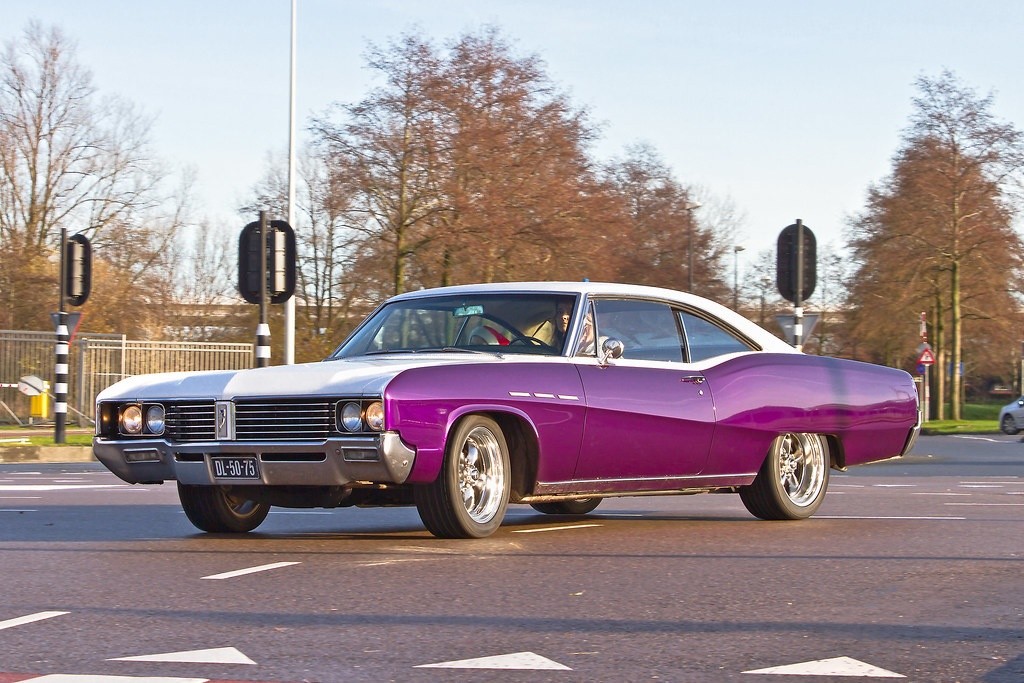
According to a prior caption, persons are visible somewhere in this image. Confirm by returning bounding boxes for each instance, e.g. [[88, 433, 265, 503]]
[[468, 325, 511, 346], [551, 296, 624, 359]]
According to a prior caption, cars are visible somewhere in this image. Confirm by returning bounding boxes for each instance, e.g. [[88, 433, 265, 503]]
[[999, 393, 1023, 436], [91, 282, 924, 541]]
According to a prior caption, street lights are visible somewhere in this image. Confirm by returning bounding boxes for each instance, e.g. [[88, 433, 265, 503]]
[[760, 276, 772, 325], [733, 244, 744, 313], [685, 200, 702, 295]]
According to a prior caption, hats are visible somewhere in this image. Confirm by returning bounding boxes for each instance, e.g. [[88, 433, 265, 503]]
[[469, 326, 510, 345]]
